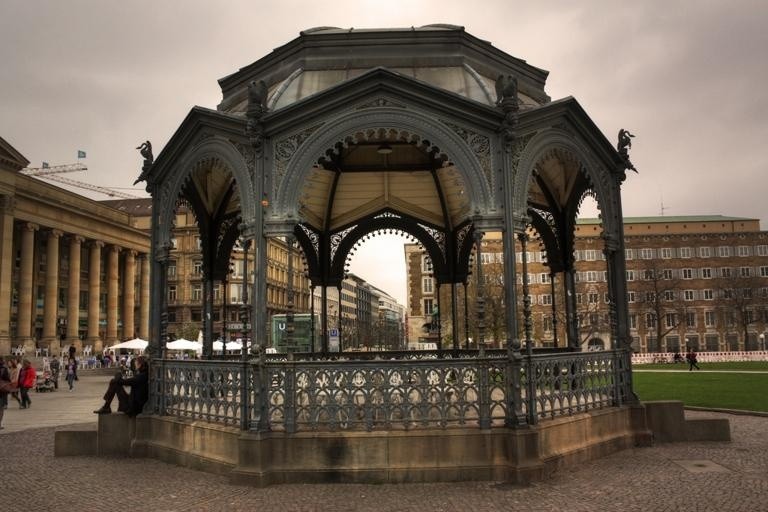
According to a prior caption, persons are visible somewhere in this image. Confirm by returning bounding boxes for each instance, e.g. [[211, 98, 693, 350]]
[[89, 350, 138, 377], [685, 351, 702, 372], [92, 355, 150, 418], [0, 343, 78, 409], [0, 356, 15, 430], [673, 350, 681, 364]]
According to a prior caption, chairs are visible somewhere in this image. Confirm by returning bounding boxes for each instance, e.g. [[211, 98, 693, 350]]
[[10, 342, 135, 377]]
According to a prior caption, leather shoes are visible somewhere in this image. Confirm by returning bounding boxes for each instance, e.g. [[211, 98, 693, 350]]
[[93, 406, 111, 413]]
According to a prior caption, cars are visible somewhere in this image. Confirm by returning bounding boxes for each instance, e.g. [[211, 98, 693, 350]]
[[12, 355, 55, 392]]
